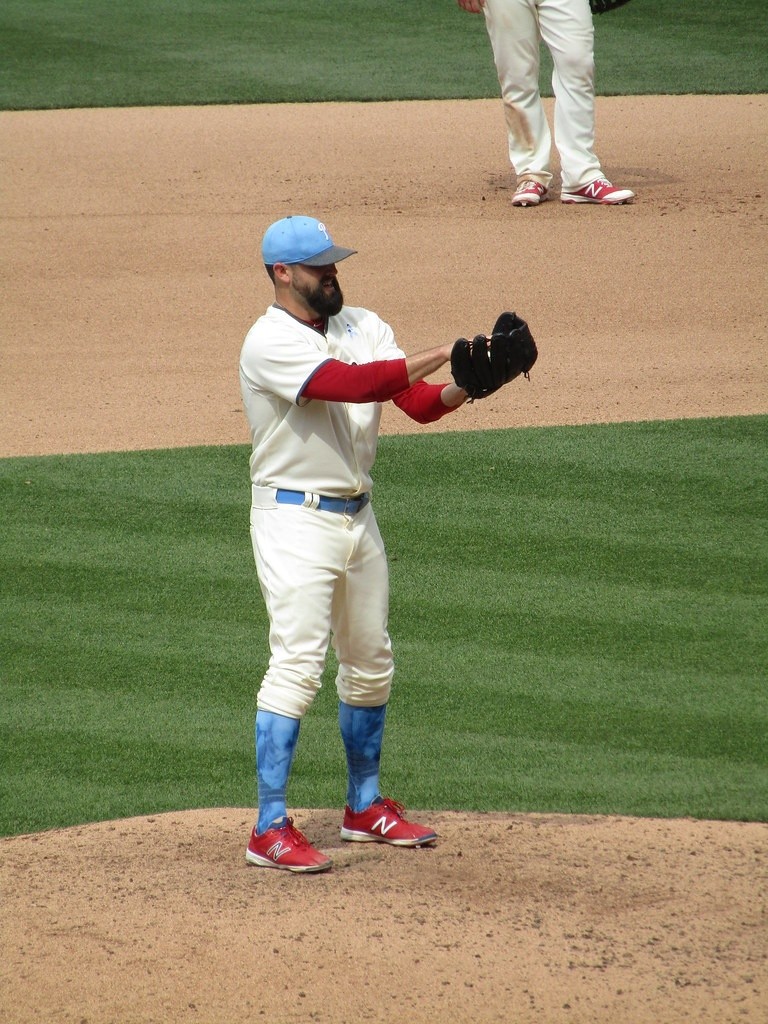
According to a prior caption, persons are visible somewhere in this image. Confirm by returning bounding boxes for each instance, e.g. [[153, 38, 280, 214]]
[[456, 0, 634, 207], [239, 217, 539, 873]]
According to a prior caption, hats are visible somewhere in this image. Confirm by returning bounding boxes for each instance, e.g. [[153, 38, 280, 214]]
[[262, 216, 359, 267]]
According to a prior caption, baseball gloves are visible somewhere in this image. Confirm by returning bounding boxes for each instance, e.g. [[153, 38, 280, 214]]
[[447, 309, 539, 406]]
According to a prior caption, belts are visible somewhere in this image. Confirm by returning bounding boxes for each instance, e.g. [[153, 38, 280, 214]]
[[276, 488, 370, 515]]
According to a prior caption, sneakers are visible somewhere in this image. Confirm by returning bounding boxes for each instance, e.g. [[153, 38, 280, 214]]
[[511, 179, 550, 206], [560, 179, 634, 205], [339, 800, 438, 847], [244, 818, 334, 872]]
